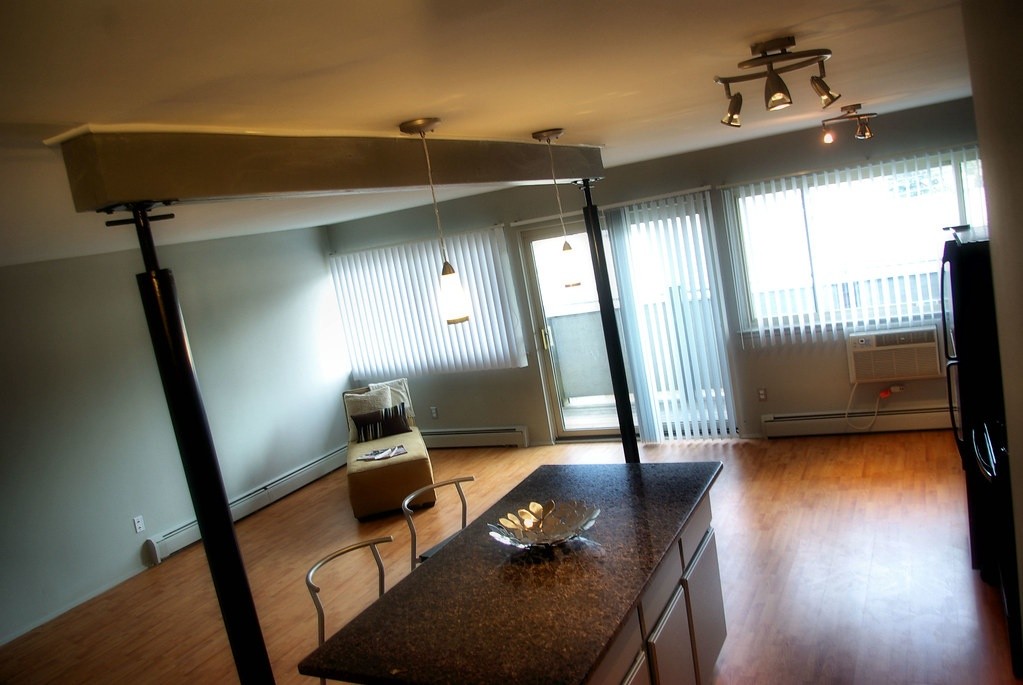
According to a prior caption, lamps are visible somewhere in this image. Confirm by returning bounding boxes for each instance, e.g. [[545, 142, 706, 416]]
[[822, 103, 879, 142], [532, 129, 583, 287], [711, 34, 843, 128], [399, 119, 471, 328]]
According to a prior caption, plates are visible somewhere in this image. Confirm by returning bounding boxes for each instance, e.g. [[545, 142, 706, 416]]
[[486, 500, 600, 550]]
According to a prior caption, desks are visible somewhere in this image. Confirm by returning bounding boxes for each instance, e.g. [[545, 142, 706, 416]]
[[298, 460, 733, 684]]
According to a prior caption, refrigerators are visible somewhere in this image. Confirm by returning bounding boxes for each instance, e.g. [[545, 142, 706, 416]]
[[939, 224, 1017, 585]]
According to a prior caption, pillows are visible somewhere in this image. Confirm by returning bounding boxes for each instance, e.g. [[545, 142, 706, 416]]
[[343, 386, 392, 444], [350, 402, 413, 443]]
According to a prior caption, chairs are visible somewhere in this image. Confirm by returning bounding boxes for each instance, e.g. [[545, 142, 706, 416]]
[[342, 378, 439, 521], [305, 535, 396, 684], [401, 476, 477, 573]]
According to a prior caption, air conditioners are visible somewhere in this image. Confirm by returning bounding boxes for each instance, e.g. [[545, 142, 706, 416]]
[[844, 326, 940, 384]]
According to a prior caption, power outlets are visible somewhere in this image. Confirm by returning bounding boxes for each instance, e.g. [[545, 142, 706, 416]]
[[134, 515, 144, 533], [758, 387, 769, 401], [891, 386, 905, 393], [430, 405, 439, 422]]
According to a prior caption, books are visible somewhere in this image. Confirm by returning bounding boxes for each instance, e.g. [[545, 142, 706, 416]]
[[356, 445, 408, 462]]
